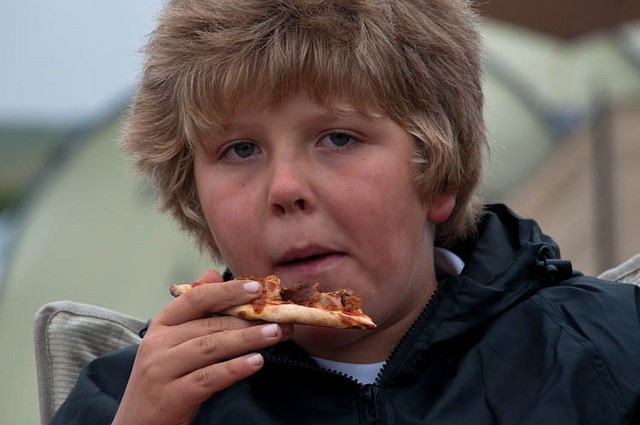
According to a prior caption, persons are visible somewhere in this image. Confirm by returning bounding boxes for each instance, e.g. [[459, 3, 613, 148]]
[[49, 2, 640, 425]]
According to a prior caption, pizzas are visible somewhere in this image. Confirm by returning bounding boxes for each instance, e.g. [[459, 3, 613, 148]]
[[170, 274, 377, 335]]
